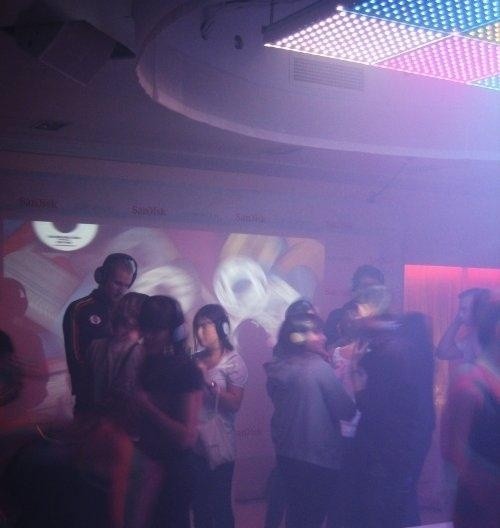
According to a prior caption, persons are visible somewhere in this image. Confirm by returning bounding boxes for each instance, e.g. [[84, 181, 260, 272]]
[[56, 290, 150, 528], [1, 269, 69, 432], [64, 252, 137, 412], [440, 298, 500, 528], [133, 297, 204, 528], [0, 328, 21, 408], [191, 305, 244, 527], [437, 284, 490, 365], [266, 262, 432, 527]]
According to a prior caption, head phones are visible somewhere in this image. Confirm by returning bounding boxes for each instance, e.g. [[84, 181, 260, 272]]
[[87, 309, 104, 329], [198, 306, 231, 339], [156, 298, 190, 344], [1, 277, 28, 319], [285, 314, 305, 347], [93, 253, 138, 290], [115, 293, 139, 326]]
